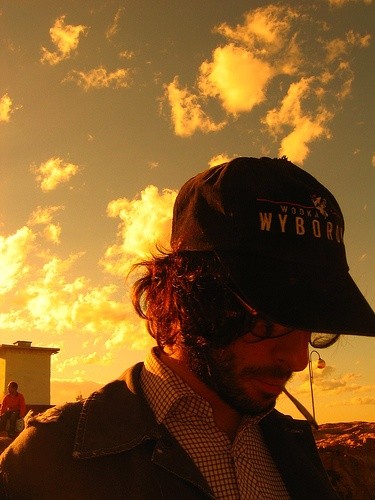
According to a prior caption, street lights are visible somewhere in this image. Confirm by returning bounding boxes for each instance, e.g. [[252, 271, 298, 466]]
[[308, 350, 327, 422]]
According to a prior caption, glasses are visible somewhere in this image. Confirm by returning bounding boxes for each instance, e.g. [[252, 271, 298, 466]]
[[169, 249, 341, 348]]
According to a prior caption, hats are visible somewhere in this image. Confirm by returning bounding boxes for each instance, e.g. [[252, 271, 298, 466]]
[[171, 156, 375, 336]]
